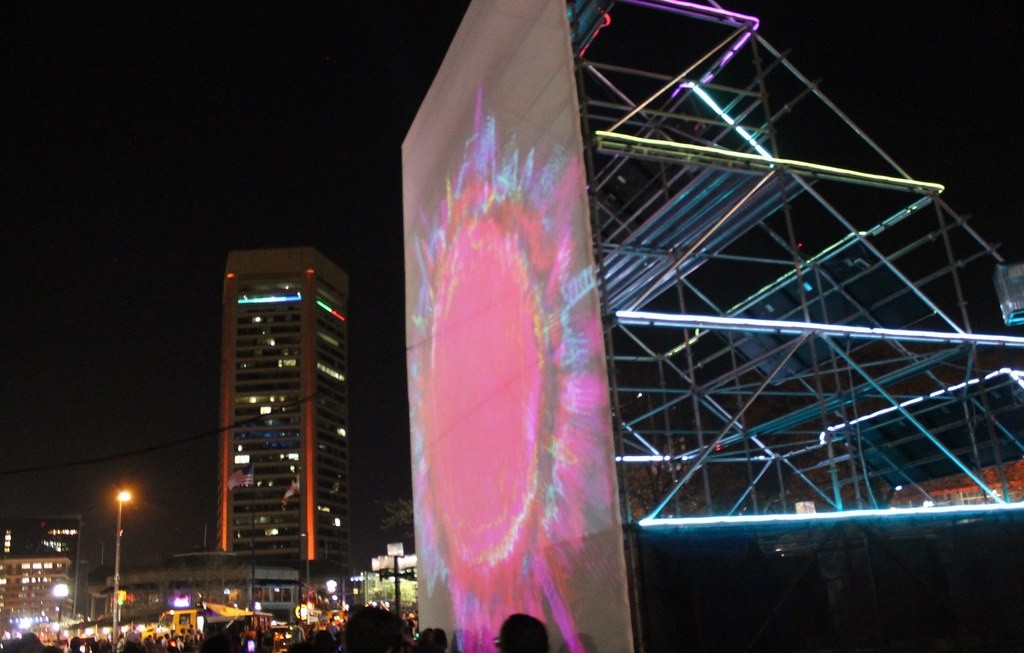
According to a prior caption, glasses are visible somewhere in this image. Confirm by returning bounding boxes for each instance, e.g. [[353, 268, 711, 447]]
[[494, 637, 502, 647]]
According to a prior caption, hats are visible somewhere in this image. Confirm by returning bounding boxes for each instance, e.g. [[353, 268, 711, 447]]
[[128, 632, 140, 643]]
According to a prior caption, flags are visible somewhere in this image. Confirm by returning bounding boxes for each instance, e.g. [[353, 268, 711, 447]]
[[228, 465, 253, 491], [284, 473, 299, 499]]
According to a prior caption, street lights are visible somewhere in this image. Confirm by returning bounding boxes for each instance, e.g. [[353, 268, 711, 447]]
[[53, 583, 70, 642], [113, 491, 131, 652], [371, 542, 417, 618]]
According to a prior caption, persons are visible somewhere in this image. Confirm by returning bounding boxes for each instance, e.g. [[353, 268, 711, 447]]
[[0, 603, 552, 653]]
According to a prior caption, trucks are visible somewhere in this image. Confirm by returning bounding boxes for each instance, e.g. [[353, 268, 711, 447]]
[[140, 608, 272, 645]]
